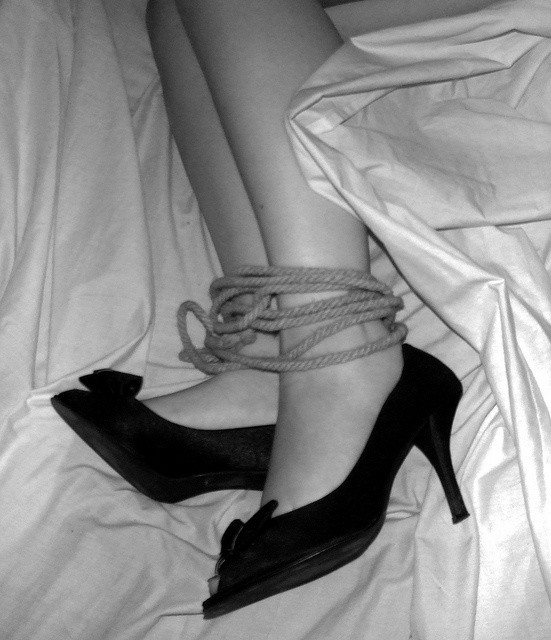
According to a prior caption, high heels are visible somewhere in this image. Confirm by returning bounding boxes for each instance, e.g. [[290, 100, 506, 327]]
[[50, 369, 275, 504], [202, 344, 470, 620]]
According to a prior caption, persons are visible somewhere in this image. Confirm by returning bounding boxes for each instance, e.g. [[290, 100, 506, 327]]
[[50, 0, 507, 618]]
